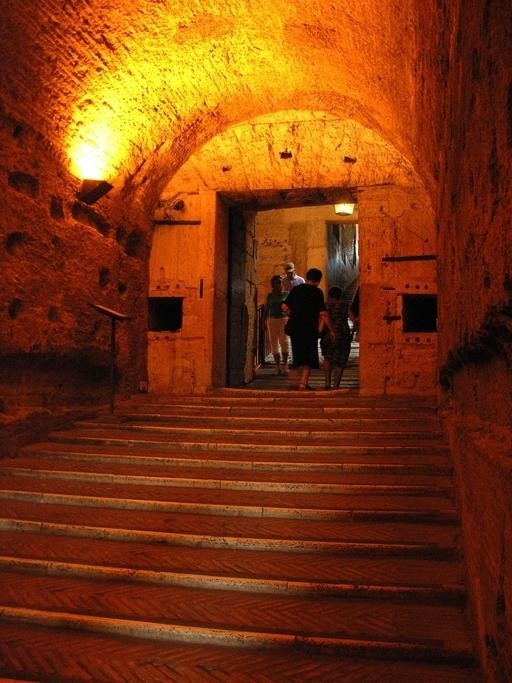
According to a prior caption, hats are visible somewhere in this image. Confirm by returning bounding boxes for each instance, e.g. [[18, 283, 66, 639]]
[[284, 262, 296, 273]]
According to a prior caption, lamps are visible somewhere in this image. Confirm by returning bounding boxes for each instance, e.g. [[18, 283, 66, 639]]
[[333, 203, 355, 216]]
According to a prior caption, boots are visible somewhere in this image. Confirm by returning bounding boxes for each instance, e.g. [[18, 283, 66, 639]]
[[272, 351, 291, 374]]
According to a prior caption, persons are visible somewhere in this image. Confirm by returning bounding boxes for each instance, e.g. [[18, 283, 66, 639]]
[[281, 262, 305, 289], [349, 285, 360, 332], [280, 268, 336, 389], [317, 285, 351, 389], [260, 273, 295, 375]]
